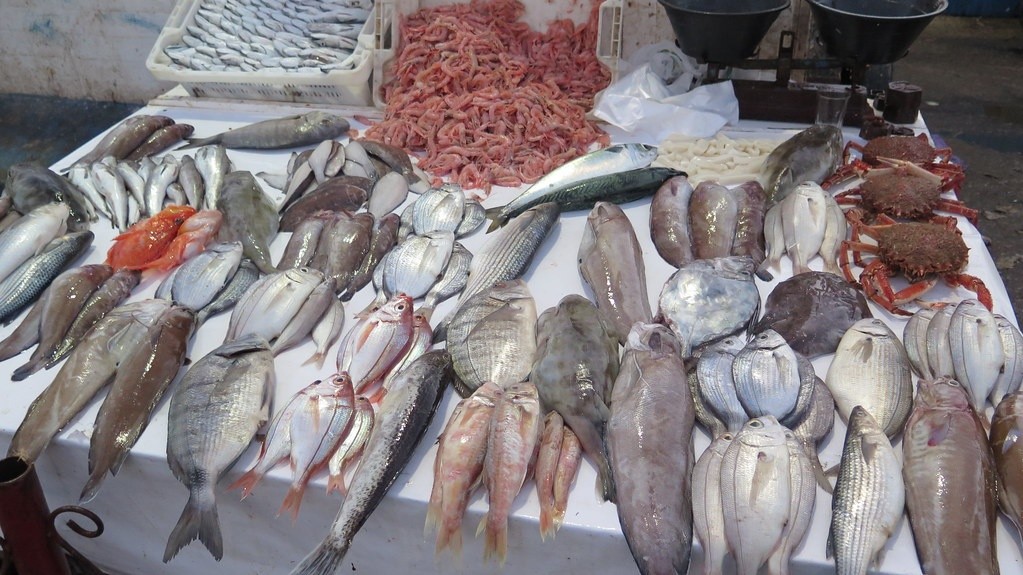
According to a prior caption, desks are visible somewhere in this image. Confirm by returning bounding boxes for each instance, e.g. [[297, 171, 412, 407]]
[[1, 86, 1023, 575]]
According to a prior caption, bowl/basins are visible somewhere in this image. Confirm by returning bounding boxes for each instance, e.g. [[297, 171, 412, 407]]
[[657, 0, 792, 56], [807, 0, 949, 59]]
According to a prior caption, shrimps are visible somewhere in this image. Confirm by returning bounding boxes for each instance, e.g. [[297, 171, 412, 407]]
[[350, 0, 612, 193]]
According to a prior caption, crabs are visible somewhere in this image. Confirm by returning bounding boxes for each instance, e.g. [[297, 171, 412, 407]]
[[822, 132, 994, 314]]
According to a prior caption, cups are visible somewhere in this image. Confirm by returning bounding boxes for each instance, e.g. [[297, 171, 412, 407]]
[[815, 86, 852, 129]]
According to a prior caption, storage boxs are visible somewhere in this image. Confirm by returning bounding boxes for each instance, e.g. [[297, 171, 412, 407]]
[[147, 0, 625, 113]]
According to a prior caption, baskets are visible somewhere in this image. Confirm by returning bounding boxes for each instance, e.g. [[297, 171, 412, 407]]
[[372, 0, 624, 111], [146, 0, 391, 107]]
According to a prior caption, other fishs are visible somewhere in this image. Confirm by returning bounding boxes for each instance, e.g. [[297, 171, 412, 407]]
[[0, 0, 1022, 575]]
